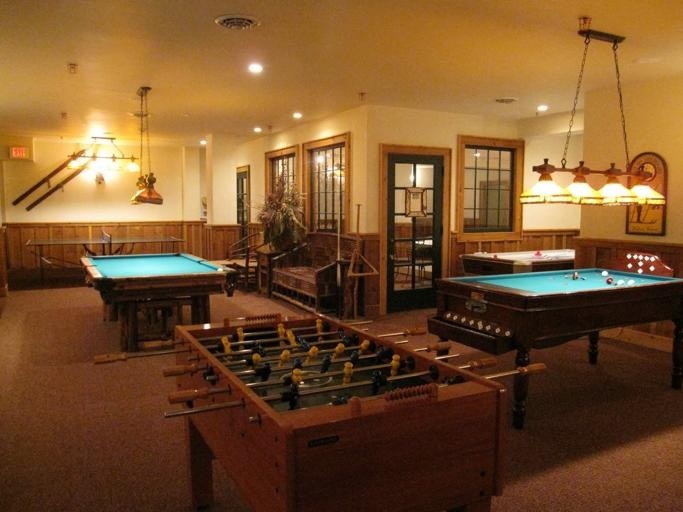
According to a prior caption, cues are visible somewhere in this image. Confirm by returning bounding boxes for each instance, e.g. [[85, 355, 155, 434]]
[[337, 204, 342, 320]]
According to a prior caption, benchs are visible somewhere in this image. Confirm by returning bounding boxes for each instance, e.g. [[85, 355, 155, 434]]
[[269, 232, 365, 314]]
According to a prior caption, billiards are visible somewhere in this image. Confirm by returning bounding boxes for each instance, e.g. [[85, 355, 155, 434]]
[[573, 270, 635, 285]]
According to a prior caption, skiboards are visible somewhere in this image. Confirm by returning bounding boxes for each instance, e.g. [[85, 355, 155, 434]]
[[12, 150, 98, 211]]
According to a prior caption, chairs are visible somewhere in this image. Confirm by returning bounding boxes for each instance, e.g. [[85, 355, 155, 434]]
[[228, 231, 264, 289], [394, 236, 433, 282]]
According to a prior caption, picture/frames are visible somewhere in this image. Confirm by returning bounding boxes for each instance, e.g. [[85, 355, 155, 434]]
[[623, 151, 667, 237]]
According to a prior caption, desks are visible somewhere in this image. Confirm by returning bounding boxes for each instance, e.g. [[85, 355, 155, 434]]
[[425, 268, 682, 430], [24, 230, 186, 286], [78, 252, 236, 353], [458, 248, 576, 274]]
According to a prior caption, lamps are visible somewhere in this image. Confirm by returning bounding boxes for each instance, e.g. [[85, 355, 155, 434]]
[[519, 27, 667, 207], [130, 86, 164, 206]]
